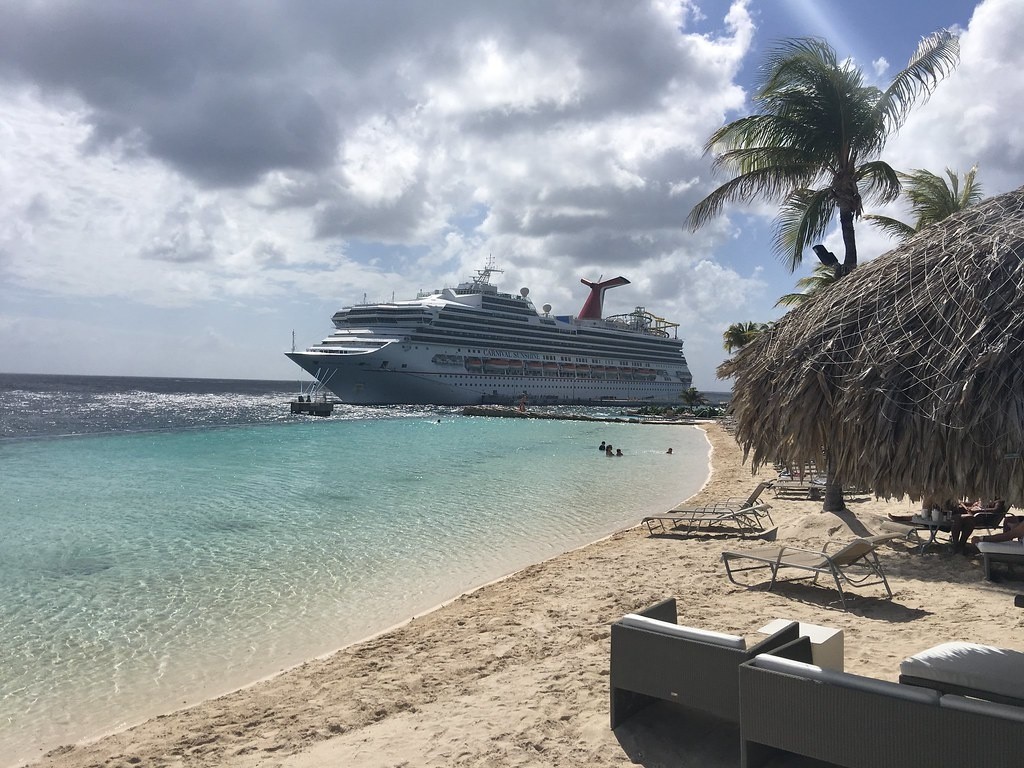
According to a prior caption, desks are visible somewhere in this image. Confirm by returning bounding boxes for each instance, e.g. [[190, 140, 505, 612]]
[[757, 619, 845, 672], [911, 514, 953, 558]]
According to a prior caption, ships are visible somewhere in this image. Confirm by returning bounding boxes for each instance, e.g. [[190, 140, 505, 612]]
[[284, 254, 693, 404]]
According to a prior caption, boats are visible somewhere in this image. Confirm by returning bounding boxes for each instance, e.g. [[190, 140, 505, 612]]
[[291, 402, 332, 415]]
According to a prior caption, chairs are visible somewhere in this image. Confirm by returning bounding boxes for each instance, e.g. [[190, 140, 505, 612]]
[[974, 540, 1024, 581], [878, 495, 1017, 557], [721, 532, 904, 611], [641, 479, 786, 536], [768, 448, 885, 501]]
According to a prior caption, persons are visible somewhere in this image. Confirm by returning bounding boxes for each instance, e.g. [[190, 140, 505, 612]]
[[887, 490, 1024, 554], [599, 440, 605, 450], [615, 449, 623, 456], [666, 448, 673, 454], [605, 445, 614, 456]]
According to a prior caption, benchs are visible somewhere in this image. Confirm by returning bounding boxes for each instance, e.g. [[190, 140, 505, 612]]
[[610, 599, 799, 731], [740, 635, 1024, 768], [899, 638, 1024, 702]]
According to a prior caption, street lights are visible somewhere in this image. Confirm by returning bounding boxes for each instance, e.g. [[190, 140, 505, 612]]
[[572, 377, 578, 404]]
